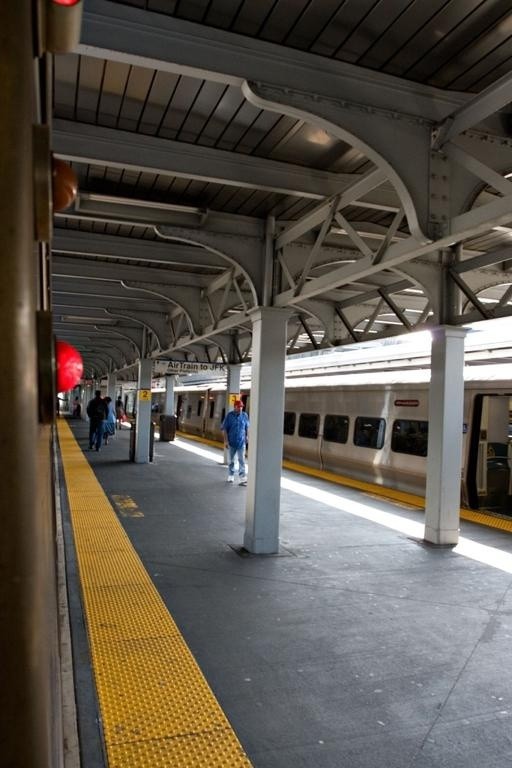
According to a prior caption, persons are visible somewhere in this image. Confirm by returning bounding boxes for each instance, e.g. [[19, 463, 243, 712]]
[[86, 389, 109, 451], [117, 400, 127, 429], [220, 400, 249, 487], [72, 397, 79, 415], [116, 395, 123, 429], [103, 396, 116, 445]]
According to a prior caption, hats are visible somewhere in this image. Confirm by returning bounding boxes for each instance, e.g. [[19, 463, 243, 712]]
[[234, 401, 245, 408]]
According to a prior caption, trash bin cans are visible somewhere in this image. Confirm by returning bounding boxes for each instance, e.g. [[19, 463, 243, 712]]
[[129, 421, 154, 462], [160, 415, 176, 441]]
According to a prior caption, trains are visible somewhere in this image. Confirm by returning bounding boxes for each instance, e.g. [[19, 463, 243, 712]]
[[124, 379, 511, 514]]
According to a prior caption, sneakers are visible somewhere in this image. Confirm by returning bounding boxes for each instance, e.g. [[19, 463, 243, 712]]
[[239, 476, 248, 485], [227, 475, 234, 482]]
